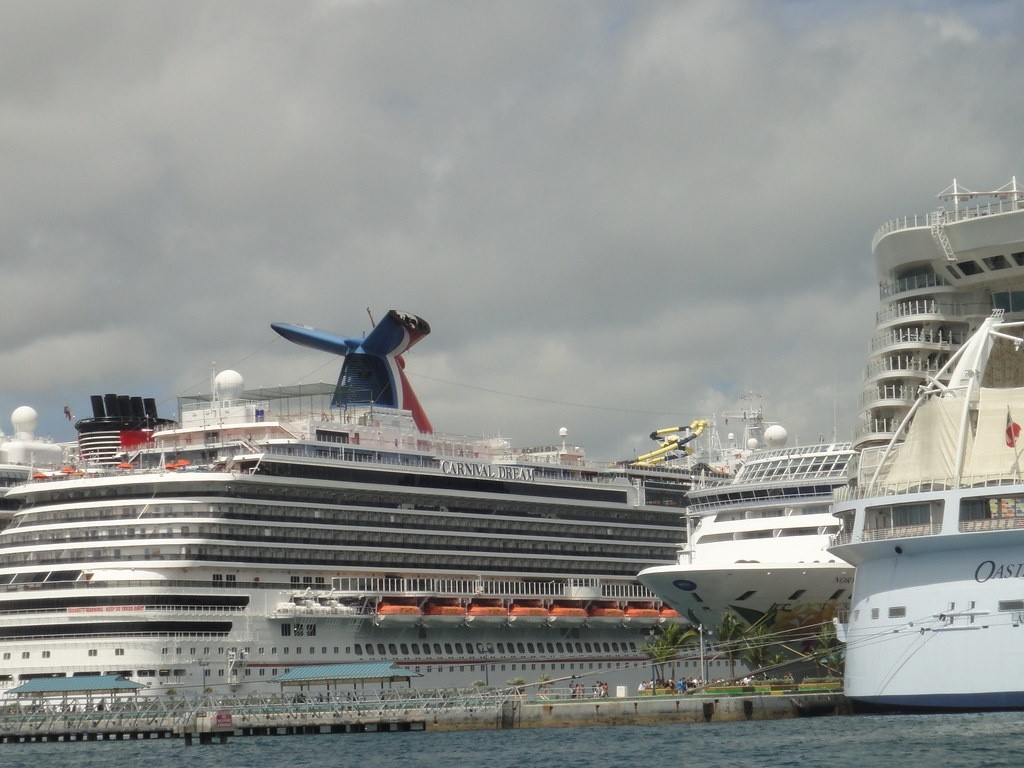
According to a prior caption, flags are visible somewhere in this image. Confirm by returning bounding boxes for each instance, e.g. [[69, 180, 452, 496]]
[[1006, 405, 1021, 448]]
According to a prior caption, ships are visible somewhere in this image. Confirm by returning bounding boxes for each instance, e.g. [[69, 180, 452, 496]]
[[1, 307, 734, 709], [827, 176, 1024, 711]]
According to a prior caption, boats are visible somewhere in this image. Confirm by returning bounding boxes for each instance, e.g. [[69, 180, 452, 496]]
[[634, 409, 857, 680]]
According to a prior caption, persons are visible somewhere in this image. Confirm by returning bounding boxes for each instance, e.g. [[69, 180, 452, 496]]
[[346, 692, 358, 701], [569, 680, 610, 699], [637, 672, 794, 695]]
